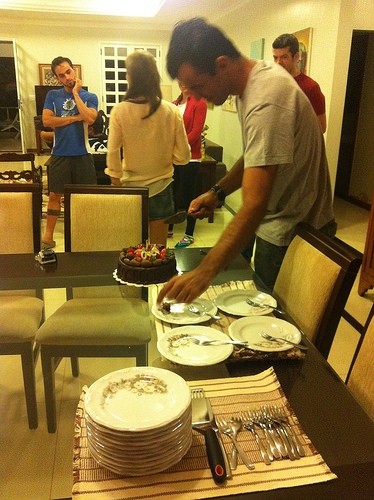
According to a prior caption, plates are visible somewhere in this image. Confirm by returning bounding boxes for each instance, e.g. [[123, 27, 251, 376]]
[[213, 289, 277, 317], [228, 315, 302, 353], [84, 365, 193, 478], [151, 297, 218, 324], [157, 326, 233, 366]]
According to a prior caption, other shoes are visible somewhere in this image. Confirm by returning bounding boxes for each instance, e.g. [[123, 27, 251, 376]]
[[41, 240, 55, 249], [174, 235, 195, 248]]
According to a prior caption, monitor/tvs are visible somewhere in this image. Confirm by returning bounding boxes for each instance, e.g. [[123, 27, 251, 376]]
[[35, 85, 88, 115]]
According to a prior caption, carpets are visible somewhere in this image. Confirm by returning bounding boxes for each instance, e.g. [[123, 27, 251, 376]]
[[41, 169, 65, 219]]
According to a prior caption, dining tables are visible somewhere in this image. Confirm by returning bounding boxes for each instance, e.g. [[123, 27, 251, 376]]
[[0, 246, 374, 500]]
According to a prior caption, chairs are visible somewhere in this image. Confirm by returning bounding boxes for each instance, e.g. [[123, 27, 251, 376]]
[[0, 183, 47, 429], [0, 152, 43, 201], [89, 109, 109, 151], [173, 160, 218, 224], [1, 112, 21, 140], [34, 183, 154, 435], [272, 221, 366, 360], [344, 302, 374, 426]]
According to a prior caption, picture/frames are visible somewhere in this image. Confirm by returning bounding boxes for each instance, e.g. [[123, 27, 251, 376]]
[[38, 63, 82, 87]]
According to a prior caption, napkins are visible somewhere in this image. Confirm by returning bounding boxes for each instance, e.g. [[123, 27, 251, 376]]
[[152, 278, 307, 367], [70, 366, 338, 500]]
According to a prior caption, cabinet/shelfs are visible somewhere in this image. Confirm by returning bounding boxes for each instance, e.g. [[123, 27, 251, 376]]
[[34, 116, 53, 157]]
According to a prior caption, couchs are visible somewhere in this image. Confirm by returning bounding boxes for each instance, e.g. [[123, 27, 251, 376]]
[[206, 138, 227, 208]]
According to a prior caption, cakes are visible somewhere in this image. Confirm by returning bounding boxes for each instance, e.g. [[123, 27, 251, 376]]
[[117, 243, 177, 284]]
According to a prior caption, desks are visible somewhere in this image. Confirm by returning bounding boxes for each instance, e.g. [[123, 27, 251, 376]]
[[0, 106, 18, 133]]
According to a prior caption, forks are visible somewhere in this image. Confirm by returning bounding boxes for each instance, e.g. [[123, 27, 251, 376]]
[[187, 305, 223, 320], [257, 331, 307, 350], [246, 298, 286, 314], [191, 338, 249, 346], [215, 404, 305, 470]]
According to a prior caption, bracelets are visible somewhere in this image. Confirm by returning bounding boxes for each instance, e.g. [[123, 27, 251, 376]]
[[211, 185, 225, 208]]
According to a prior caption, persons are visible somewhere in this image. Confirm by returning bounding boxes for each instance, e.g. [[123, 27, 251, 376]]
[[172, 78, 207, 248], [104, 51, 191, 246], [41, 116, 108, 185], [157, 17, 338, 305], [40, 57, 98, 250], [271, 34, 327, 134]]
